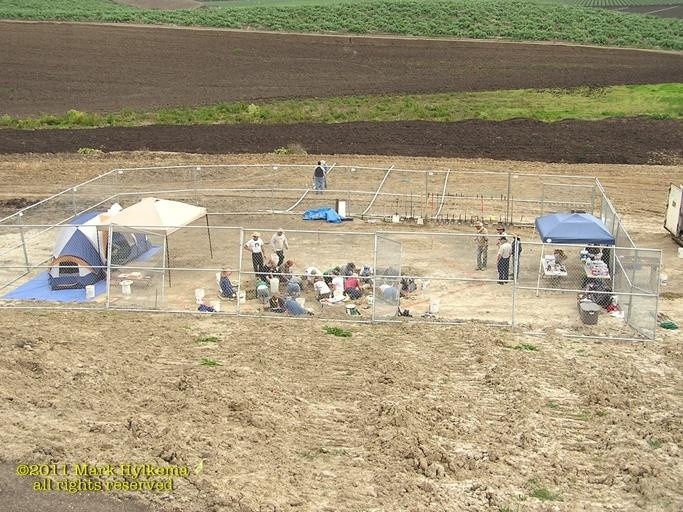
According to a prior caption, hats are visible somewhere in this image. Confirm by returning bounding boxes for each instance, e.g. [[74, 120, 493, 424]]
[[221, 267, 232, 277], [252, 232, 261, 237], [497, 225, 505, 230], [474, 222, 483, 226]]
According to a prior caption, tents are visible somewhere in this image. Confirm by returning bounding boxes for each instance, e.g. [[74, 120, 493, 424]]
[[48, 212, 147, 290]]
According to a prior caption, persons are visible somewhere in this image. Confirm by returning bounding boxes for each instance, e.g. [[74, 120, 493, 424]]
[[313, 161, 326, 194], [322, 160, 329, 173], [216, 228, 418, 316], [475, 220, 522, 285]]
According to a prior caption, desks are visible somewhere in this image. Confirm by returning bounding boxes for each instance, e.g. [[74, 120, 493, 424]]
[[109, 271, 153, 290], [541, 256, 611, 294]]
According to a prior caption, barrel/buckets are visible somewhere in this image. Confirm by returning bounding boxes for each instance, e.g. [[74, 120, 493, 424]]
[[345, 304, 357, 315], [120, 280, 133, 294], [86, 285, 94, 297], [237, 291, 246, 304], [392, 215, 399, 224], [417, 218, 423, 225], [270, 278, 279, 293], [430, 299, 439, 313], [194, 289, 205, 303], [295, 298, 306, 307]]
